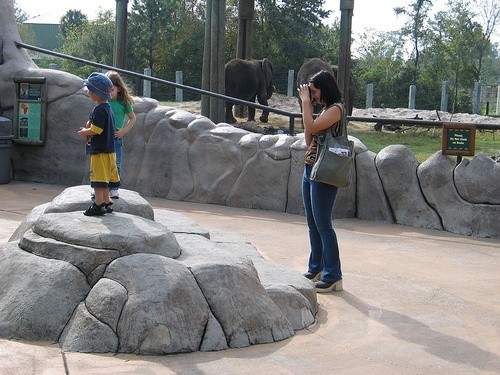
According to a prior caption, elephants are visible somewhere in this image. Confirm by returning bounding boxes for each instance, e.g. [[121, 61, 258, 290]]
[[297, 58, 356, 123], [224, 58, 277, 123]]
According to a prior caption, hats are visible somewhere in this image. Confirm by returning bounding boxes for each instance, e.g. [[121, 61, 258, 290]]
[[82, 72, 113, 99]]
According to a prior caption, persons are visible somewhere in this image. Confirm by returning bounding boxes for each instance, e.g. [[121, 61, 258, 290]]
[[296, 70, 348, 294], [89, 70, 136, 199], [78, 71, 121, 216]]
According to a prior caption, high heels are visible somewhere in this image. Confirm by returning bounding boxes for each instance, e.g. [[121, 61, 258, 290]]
[[315, 276, 343, 293], [303, 271, 322, 282]]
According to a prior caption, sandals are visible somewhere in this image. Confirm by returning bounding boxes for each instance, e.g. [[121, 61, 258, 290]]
[[84, 202, 113, 215]]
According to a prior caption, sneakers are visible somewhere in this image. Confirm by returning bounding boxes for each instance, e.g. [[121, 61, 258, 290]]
[[91, 189, 95, 198], [110, 189, 120, 199]]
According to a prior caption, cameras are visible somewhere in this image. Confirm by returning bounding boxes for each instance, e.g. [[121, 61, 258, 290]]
[[308, 86, 312, 100]]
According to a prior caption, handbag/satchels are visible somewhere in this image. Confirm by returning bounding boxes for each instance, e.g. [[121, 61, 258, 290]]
[[310, 103, 355, 188]]
